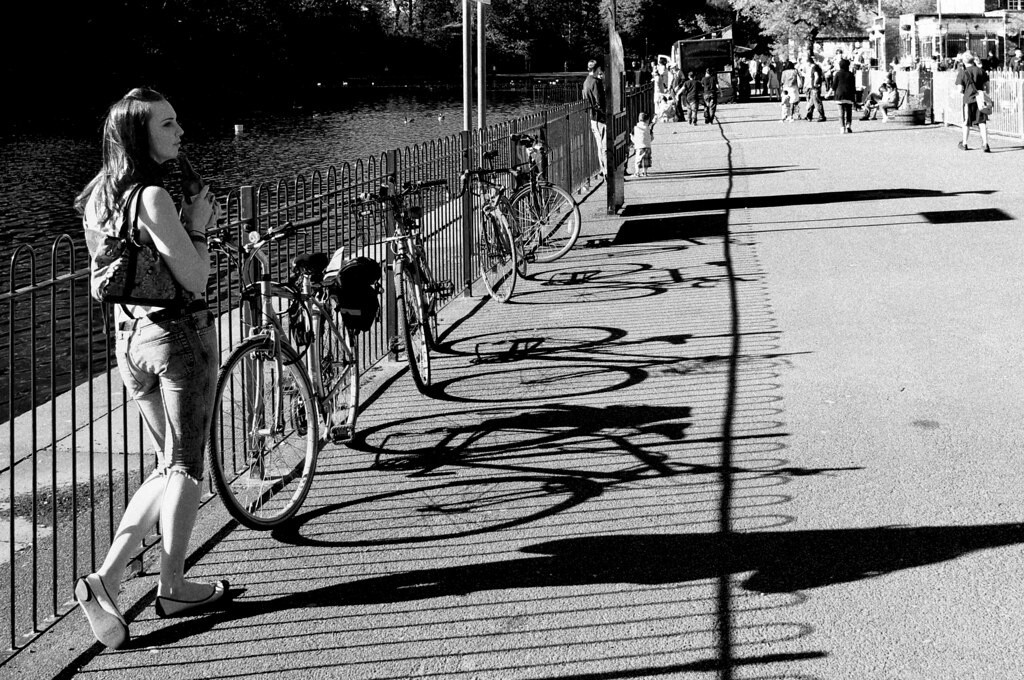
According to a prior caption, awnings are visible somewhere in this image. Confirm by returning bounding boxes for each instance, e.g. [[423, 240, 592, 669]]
[[734, 45, 755, 60]]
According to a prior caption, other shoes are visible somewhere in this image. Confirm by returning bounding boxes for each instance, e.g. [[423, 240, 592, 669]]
[[882, 116, 888, 124], [817, 116, 826, 122], [74, 573, 129, 650], [641, 173, 647, 177], [630, 174, 640, 177], [982, 143, 990, 152], [155, 579, 229, 619], [846, 124, 852, 133], [859, 116, 869, 120], [958, 141, 967, 150], [840, 127, 846, 134], [806, 115, 812, 122]]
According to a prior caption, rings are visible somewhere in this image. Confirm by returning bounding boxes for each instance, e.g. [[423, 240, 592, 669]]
[[207, 194, 215, 202]]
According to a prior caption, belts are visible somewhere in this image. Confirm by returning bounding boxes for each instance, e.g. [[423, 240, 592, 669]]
[[119, 298, 210, 331]]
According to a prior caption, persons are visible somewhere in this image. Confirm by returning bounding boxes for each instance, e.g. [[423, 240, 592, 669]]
[[755, 49, 866, 133], [584, 60, 632, 181], [988, 51, 999, 70], [651, 61, 719, 125], [1006, 47, 1011, 71], [892, 52, 918, 72], [736, 61, 753, 100], [631, 113, 654, 177], [630, 62, 639, 90], [859, 73, 898, 123], [1009, 48, 1024, 78], [929, 51, 991, 152], [74, 86, 231, 650]]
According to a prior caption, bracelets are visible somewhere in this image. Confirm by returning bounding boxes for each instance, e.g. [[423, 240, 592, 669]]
[[189, 230, 207, 243]]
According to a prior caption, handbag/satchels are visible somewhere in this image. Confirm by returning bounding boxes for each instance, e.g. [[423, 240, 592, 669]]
[[85, 181, 187, 308], [975, 90, 993, 115]]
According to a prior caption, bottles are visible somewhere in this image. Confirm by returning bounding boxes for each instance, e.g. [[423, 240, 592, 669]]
[[177, 153, 203, 205]]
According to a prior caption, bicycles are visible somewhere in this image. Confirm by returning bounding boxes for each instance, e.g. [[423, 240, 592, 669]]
[[350, 178, 456, 394], [508, 131, 582, 264], [456, 147, 529, 305], [204, 215, 360, 531]]
